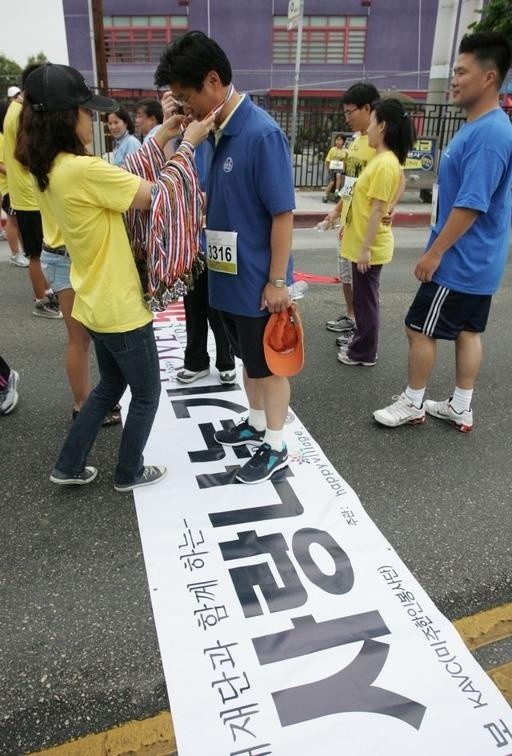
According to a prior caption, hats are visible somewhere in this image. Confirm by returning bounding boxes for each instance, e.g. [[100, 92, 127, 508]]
[[262, 300, 304, 377], [24, 62, 119, 114], [7, 87, 21, 98]]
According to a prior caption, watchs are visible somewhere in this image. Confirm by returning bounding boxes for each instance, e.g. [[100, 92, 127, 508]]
[[268, 278, 286, 288]]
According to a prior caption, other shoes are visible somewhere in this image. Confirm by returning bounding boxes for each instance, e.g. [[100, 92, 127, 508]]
[[71, 408, 121, 427], [113, 404, 121, 412]]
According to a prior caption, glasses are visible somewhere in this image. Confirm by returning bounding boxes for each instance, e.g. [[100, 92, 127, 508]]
[[343, 105, 360, 118]]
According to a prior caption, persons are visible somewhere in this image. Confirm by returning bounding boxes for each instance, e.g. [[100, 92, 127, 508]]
[[0, 62, 236, 426], [14, 64, 217, 493], [310, 83, 382, 347], [322, 133, 347, 202], [335, 98, 418, 368], [152, 28, 297, 487], [370, 30, 510, 433]]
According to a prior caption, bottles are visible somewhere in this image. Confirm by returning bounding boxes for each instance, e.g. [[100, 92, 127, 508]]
[[264, 278, 309, 308], [313, 217, 341, 232]]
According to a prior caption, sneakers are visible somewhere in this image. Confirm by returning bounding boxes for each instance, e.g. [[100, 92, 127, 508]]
[[214, 417, 266, 446], [2, 368, 19, 415], [114, 465, 169, 490], [235, 442, 288, 484], [326, 314, 473, 433], [220, 368, 237, 384], [45, 292, 59, 305], [32, 296, 63, 319], [9, 252, 30, 268], [176, 367, 210, 384], [49, 466, 98, 484]]
